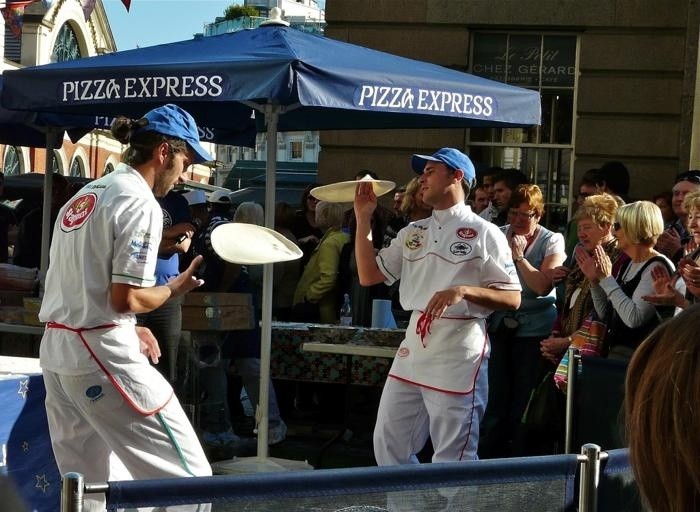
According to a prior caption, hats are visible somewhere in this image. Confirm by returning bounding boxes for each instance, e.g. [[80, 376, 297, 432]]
[[208, 190, 231, 204], [135, 104, 213, 164], [674, 170, 700, 185], [411, 147, 476, 190], [183, 191, 206, 205]]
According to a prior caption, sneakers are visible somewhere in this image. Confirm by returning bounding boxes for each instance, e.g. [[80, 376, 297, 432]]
[[269, 419, 287, 444]]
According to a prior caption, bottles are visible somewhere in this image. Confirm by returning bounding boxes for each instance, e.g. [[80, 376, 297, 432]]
[[340, 292, 355, 328], [32, 269, 41, 297]]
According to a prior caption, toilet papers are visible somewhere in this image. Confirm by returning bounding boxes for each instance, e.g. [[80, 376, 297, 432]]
[[372, 299, 398, 328]]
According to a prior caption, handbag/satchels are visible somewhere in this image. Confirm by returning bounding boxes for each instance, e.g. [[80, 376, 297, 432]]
[[275, 301, 319, 323], [553, 319, 606, 397]]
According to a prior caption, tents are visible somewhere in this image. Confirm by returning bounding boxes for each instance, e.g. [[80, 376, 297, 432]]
[[1, 5, 539, 476]]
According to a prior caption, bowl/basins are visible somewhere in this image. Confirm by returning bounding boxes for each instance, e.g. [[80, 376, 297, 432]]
[[23, 297, 43, 325]]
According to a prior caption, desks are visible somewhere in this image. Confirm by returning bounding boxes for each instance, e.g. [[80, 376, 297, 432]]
[[1, 313, 191, 427], [254, 320, 410, 384]]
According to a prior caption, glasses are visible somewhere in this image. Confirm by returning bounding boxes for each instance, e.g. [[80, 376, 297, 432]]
[[614, 221, 621, 231], [508, 210, 535, 219]]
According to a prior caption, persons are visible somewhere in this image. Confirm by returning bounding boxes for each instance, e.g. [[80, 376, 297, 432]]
[[1, 105, 700, 511]]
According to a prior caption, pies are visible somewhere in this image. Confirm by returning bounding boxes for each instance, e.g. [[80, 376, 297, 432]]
[[309, 174, 396, 203], [210, 220, 304, 266]]
